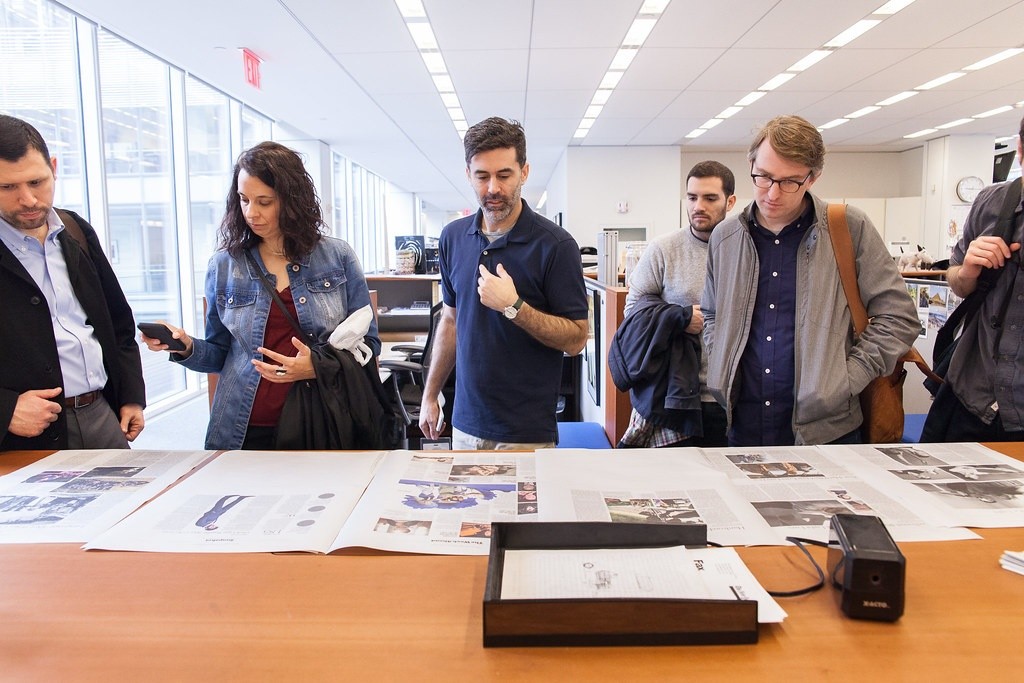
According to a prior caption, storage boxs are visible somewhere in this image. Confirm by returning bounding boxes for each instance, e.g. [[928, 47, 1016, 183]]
[[482, 518, 761, 645]]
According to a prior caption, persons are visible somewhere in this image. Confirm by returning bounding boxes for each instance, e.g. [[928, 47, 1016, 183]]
[[0, 116, 146, 450], [518, 482, 538, 514], [831, 490, 868, 511], [418, 116, 589, 448], [139, 140, 381, 450], [700, 115, 922, 447], [948, 482, 1003, 503], [195, 495, 256, 531], [379, 518, 422, 533], [623, 160, 738, 447], [919, 114, 1024, 443], [774, 463, 803, 475]]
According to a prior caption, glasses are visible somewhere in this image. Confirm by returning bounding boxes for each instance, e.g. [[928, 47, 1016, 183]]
[[751, 160, 812, 193]]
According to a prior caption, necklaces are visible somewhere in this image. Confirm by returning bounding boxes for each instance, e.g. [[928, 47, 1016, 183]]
[[272, 252, 285, 255]]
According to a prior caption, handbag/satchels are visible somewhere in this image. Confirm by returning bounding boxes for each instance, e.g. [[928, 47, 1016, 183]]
[[921, 289, 990, 397], [866, 344, 947, 444]]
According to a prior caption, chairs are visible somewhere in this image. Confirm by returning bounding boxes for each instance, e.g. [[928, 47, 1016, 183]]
[[378, 301, 445, 440]]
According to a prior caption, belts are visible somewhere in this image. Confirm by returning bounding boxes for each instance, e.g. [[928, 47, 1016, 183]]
[[65, 390, 101, 409]]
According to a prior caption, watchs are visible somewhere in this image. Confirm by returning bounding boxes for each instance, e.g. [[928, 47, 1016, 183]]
[[502, 297, 523, 320]]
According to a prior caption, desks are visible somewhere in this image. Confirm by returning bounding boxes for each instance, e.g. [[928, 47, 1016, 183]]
[[1, 448, 1024, 681]]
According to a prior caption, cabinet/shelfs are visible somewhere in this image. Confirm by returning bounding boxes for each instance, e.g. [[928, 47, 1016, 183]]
[[356, 271, 439, 358]]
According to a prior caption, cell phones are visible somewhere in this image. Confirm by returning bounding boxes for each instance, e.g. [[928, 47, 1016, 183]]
[[138, 323, 186, 351]]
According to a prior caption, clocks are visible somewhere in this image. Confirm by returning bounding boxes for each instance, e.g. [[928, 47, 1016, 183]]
[[956, 174, 986, 204]]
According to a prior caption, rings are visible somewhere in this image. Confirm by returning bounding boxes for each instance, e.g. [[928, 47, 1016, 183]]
[[275, 366, 287, 376]]
